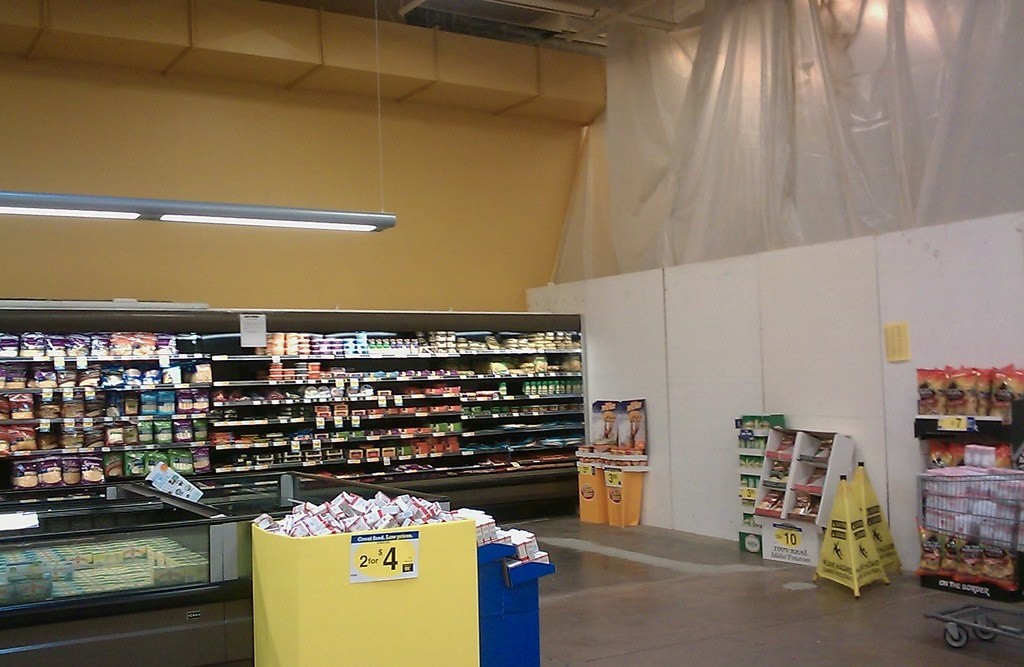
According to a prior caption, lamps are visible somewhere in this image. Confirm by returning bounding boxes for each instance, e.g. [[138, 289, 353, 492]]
[[0, 0, 397, 233]]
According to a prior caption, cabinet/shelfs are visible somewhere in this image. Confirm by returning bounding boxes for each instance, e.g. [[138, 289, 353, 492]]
[[734, 413, 784, 499], [0, 297, 586, 667], [914, 362, 1024, 602], [753, 430, 857, 528]]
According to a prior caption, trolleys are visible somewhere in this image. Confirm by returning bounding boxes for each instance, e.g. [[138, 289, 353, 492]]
[[916, 472, 1024, 648]]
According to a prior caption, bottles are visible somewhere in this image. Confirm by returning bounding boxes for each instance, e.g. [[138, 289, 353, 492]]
[[522, 381, 560, 394], [499, 382, 507, 395], [560, 380, 582, 394]]
